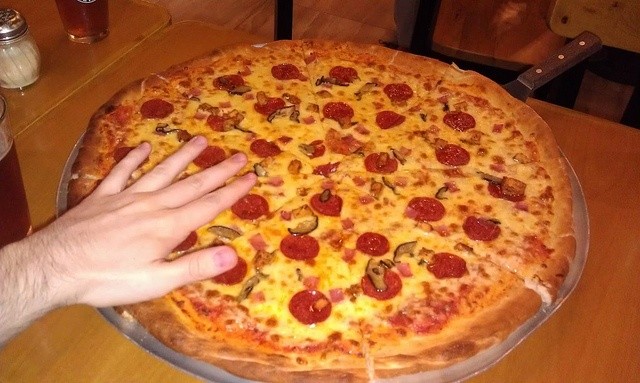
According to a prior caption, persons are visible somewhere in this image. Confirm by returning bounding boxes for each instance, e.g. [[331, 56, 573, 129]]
[[0, 130, 258, 345]]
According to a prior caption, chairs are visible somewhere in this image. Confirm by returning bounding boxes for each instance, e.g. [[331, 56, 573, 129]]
[[395, 0, 566, 100]]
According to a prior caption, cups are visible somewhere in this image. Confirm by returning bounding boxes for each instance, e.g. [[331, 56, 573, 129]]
[[1, 97, 34, 246], [55, 2, 111, 43]]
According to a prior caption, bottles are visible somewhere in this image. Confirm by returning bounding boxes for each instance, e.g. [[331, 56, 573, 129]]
[[1, 9, 43, 90]]
